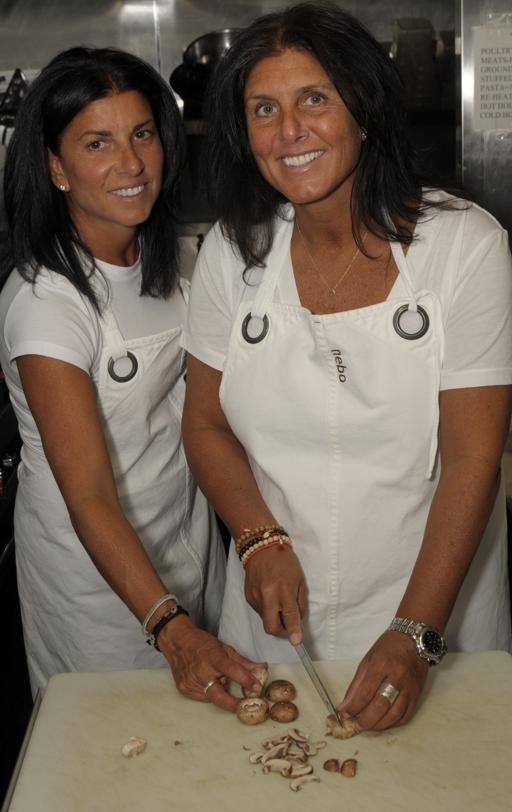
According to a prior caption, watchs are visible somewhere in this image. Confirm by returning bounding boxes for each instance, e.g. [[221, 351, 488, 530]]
[[389, 616, 448, 666]]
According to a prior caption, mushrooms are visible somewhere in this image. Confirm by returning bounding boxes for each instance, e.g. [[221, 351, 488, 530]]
[[121, 666, 361, 790]]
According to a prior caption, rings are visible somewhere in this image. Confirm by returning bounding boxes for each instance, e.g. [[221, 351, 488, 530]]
[[203, 679, 220, 696], [374, 682, 400, 706]]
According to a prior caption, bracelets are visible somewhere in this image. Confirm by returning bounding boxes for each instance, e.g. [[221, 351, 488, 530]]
[[236, 523, 293, 570], [147, 604, 190, 653], [142, 593, 179, 635]]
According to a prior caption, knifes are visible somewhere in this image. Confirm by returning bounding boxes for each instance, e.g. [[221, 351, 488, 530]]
[[279, 611, 344, 729]]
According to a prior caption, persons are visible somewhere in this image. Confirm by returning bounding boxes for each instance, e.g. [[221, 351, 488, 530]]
[[0, 45, 270, 717], [175, 0, 512, 733]]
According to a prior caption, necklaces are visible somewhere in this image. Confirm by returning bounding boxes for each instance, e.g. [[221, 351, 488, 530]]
[[294, 220, 374, 295]]
